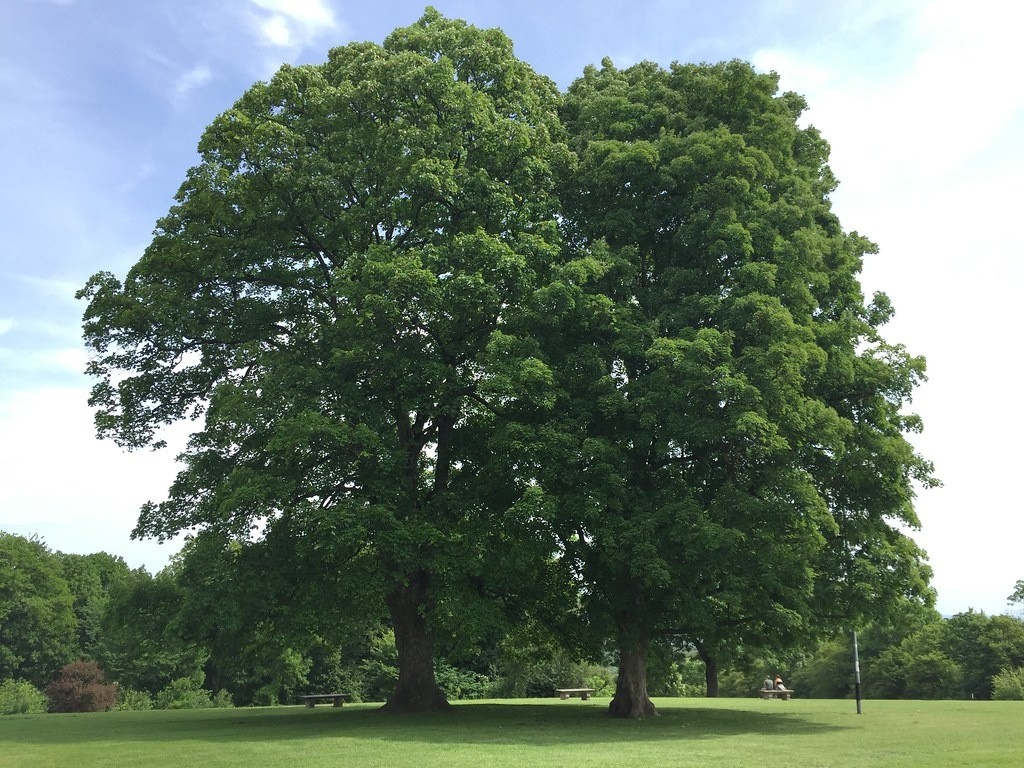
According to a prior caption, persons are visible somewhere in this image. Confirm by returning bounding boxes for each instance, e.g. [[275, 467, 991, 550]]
[[760, 676, 773, 698], [774, 674, 783, 698]]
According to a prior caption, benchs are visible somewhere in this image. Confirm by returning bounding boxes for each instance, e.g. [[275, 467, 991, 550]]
[[758, 690, 794, 700], [300, 694, 348, 707], [556, 689, 595, 701]]
